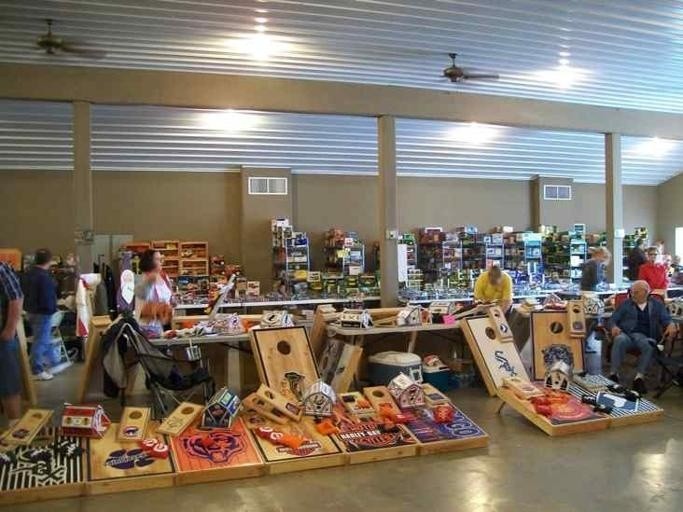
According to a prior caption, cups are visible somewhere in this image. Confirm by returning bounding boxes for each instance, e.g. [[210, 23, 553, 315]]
[[185, 345, 201, 361]]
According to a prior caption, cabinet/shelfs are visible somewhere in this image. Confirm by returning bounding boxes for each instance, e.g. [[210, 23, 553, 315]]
[[121, 228, 649, 291]]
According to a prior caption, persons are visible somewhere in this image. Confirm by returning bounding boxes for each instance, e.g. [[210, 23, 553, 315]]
[[474, 265, 514, 321], [0, 259, 23, 430], [638, 248, 670, 288], [581, 246, 610, 290], [135, 249, 178, 390], [26, 247, 61, 381], [628, 239, 682, 277], [95, 253, 117, 314], [606, 279, 678, 393]]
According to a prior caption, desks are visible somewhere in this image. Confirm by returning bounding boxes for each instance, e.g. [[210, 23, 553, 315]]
[[511, 304, 612, 330], [148, 333, 251, 397], [325, 324, 464, 383]]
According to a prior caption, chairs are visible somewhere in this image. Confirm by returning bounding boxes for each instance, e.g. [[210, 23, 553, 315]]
[[127, 324, 210, 416], [607, 293, 683, 397], [26, 311, 73, 380]]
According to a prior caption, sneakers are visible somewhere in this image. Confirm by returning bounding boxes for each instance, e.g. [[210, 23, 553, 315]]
[[609, 373, 620, 385], [32, 372, 54, 381], [629, 376, 649, 395]]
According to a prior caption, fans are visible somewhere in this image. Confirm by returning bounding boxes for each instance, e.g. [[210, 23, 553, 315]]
[[442, 54, 499, 83], [32, 19, 105, 62]]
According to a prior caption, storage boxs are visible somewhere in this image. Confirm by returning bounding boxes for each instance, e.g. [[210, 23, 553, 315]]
[[368, 351, 451, 392]]
